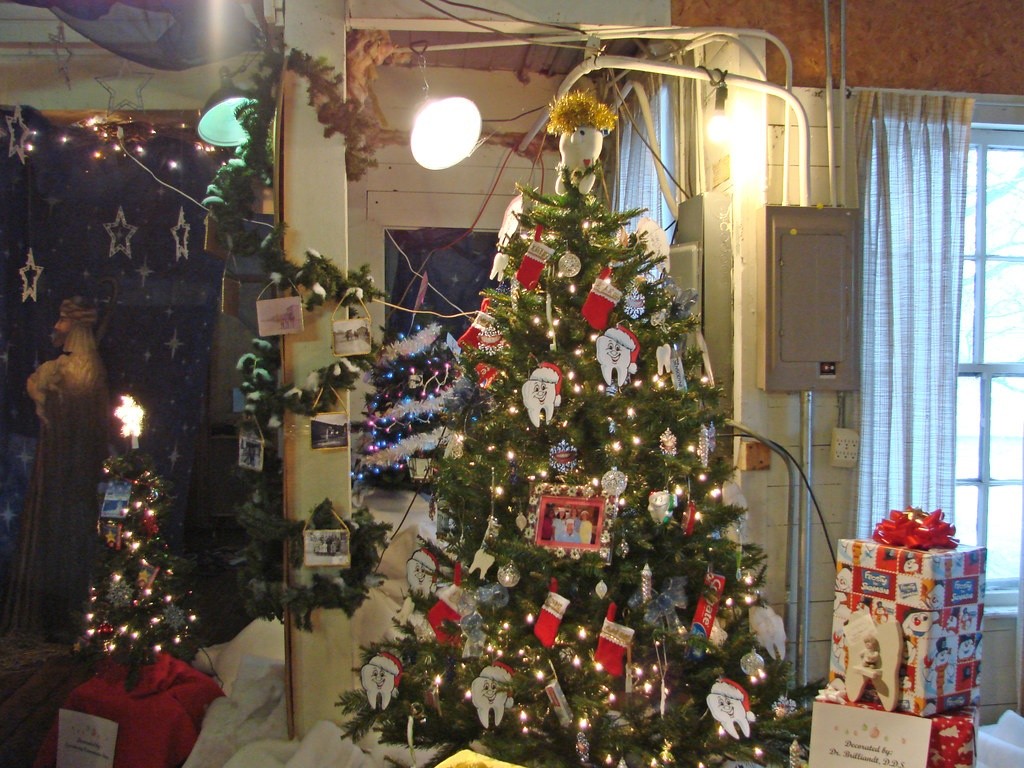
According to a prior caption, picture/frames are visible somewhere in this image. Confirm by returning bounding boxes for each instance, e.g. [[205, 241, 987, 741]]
[[518, 484, 620, 567]]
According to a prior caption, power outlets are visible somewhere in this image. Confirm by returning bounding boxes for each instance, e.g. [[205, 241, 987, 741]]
[[829, 427, 859, 469]]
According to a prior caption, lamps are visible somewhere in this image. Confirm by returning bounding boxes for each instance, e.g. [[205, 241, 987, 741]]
[[697, 65, 731, 146], [197, 45, 264, 148], [408, 39, 482, 171]]
